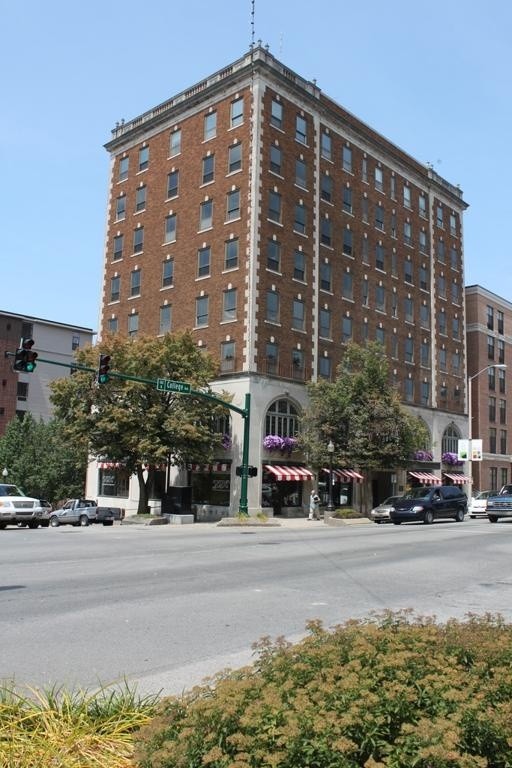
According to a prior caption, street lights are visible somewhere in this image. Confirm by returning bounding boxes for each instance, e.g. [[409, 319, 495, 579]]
[[467, 364, 507, 522]]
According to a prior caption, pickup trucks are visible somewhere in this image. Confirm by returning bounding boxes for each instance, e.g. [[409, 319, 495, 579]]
[[46, 499, 126, 528]]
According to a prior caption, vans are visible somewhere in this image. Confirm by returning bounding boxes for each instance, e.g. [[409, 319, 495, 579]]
[[390, 485, 466, 526]]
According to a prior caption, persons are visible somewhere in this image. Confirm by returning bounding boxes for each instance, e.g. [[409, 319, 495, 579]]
[[306, 489, 321, 520], [432, 492, 441, 501]]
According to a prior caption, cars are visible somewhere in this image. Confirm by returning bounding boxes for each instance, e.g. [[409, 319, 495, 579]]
[[468, 483, 512, 523], [370, 495, 403, 524], [0, 484, 43, 531]]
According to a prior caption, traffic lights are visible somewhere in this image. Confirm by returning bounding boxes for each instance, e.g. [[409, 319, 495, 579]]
[[12, 337, 38, 374], [98, 354, 110, 384]]
